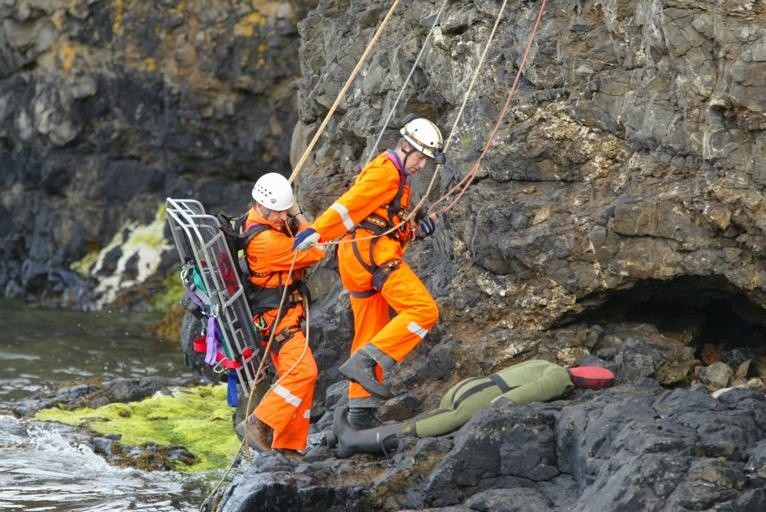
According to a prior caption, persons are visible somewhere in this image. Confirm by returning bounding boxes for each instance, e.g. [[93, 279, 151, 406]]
[[234, 172, 326, 462], [292, 117, 444, 430], [334, 359, 615, 458]]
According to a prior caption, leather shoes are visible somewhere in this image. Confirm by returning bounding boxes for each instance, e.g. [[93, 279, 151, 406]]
[[415, 215, 436, 242], [287, 197, 303, 218], [290, 228, 321, 253]]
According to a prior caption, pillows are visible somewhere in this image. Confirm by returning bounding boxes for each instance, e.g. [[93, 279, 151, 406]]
[[399, 117, 444, 159], [251, 172, 295, 212]]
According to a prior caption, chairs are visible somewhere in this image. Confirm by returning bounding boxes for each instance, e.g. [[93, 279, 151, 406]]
[[234, 412, 305, 462], [337, 348, 391, 398], [349, 408, 400, 432]]
